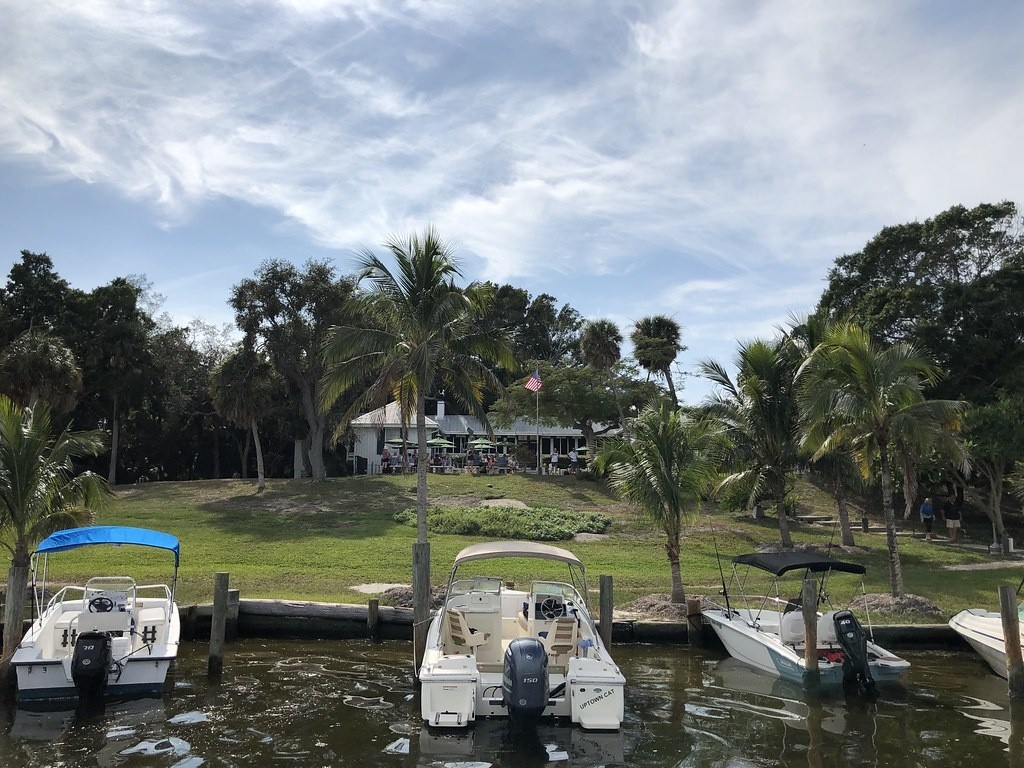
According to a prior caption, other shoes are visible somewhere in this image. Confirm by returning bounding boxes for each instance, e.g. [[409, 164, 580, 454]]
[[952, 538, 958, 543], [926, 539, 932, 542]]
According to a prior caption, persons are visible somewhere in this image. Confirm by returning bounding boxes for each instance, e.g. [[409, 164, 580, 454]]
[[568, 447, 579, 475], [466, 443, 518, 476], [382, 446, 449, 475], [549, 447, 558, 475]]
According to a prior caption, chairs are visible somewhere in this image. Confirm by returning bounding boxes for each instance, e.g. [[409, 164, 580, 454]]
[[54, 607, 166, 646], [776, 610, 849, 649], [538, 616, 579, 666], [448, 610, 490, 659]]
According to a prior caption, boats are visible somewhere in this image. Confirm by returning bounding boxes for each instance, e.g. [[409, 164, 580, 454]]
[[9, 525, 181, 701], [409, 540, 627, 731], [698, 552, 912, 691], [949, 604, 1024, 679]]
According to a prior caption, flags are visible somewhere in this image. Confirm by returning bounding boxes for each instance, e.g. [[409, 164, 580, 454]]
[[525, 368, 542, 392]]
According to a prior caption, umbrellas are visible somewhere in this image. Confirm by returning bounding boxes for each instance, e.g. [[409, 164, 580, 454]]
[[385, 436, 418, 446], [466, 437, 493, 458], [575, 445, 589, 451], [474, 445, 494, 449], [425, 437, 455, 444], [428, 444, 456, 454], [391, 443, 418, 449]]
